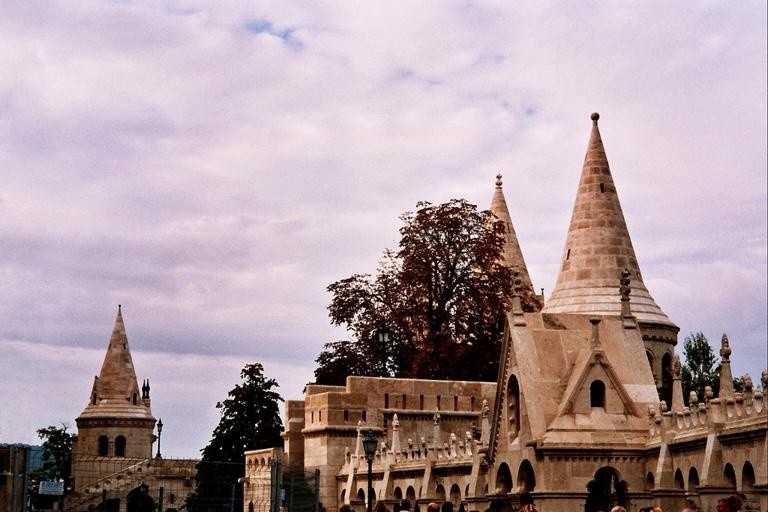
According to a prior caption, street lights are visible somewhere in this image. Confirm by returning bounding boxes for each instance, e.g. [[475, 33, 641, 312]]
[[356, 424, 384, 512], [155, 417, 163, 457]]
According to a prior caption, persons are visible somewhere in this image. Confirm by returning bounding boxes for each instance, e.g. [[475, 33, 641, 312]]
[[339, 495, 750, 510]]
